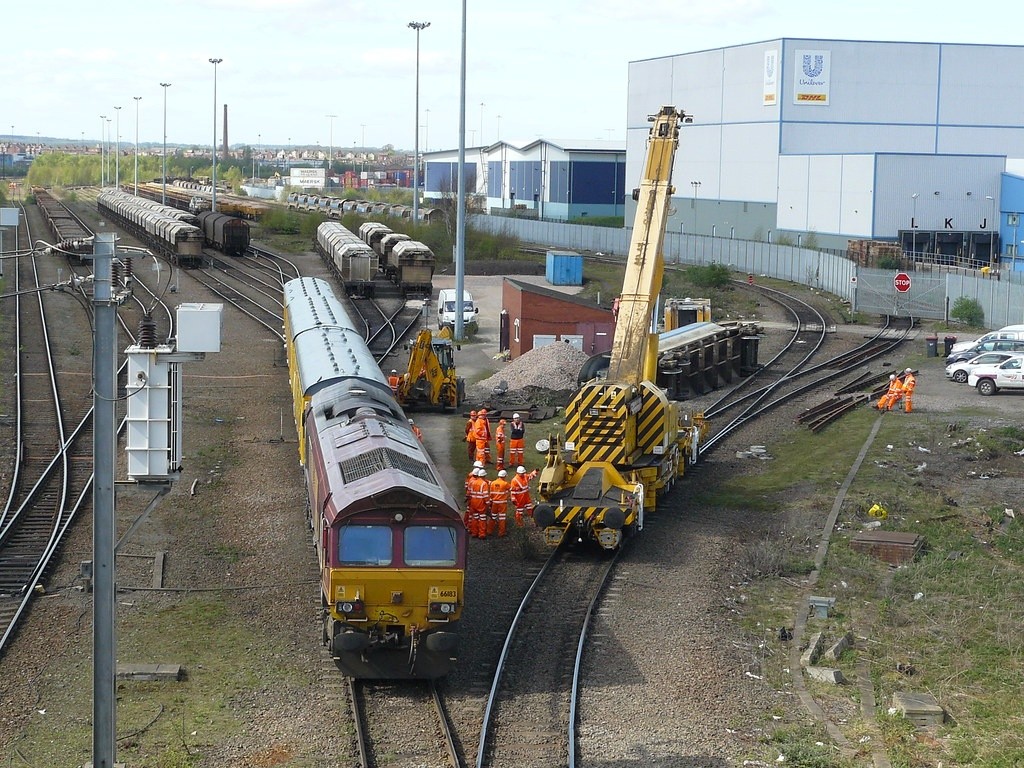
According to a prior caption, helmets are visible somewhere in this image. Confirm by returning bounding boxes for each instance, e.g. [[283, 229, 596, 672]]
[[500, 419, 506, 424], [906, 368, 911, 372], [408, 419, 414, 424], [890, 374, 896, 382], [470, 411, 476, 416], [517, 466, 527, 473], [392, 370, 396, 372], [473, 460, 483, 468], [472, 468, 487, 476], [512, 413, 519, 418], [498, 470, 508, 477], [478, 409, 487, 415]]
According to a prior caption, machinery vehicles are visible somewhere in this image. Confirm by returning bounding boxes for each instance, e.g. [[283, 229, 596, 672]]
[[393, 328, 467, 414], [532, 102, 708, 551]]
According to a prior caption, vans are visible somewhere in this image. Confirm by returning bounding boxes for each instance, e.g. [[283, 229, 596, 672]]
[[436, 288, 479, 336]]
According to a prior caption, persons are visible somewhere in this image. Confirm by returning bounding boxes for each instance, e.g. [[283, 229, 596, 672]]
[[407, 418, 422, 441], [872, 374, 903, 411], [464, 303, 472, 311], [901, 368, 916, 413], [463, 409, 542, 538], [388, 369, 399, 395]]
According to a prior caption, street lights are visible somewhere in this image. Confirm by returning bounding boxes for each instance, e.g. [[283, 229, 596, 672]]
[[911, 193, 920, 272], [133, 96, 142, 197], [208, 57, 224, 212], [160, 82, 172, 205], [986, 196, 996, 271], [99, 116, 112, 188], [114, 106, 122, 190]]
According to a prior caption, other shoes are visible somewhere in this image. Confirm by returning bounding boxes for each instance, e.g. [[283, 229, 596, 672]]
[[468, 459, 475, 464], [509, 465, 514, 468], [487, 460, 494, 464], [904, 411, 912, 414], [871, 405, 879, 410], [471, 536, 490, 540], [881, 409, 886, 414], [518, 465, 525, 467]]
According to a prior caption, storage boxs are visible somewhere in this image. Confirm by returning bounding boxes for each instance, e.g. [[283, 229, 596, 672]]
[[546, 251, 582, 286], [340, 169, 415, 188]]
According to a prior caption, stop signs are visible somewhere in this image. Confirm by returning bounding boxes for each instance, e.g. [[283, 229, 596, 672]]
[[894, 272, 912, 292]]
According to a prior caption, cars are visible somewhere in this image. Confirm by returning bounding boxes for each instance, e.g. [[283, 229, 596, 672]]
[[945, 351, 1024, 384], [968, 356, 1024, 396], [945, 325, 1024, 366]]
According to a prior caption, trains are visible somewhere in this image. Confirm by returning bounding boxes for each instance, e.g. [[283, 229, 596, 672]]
[[358, 223, 436, 299], [314, 221, 380, 298], [97, 193, 204, 269], [196, 211, 251, 255], [100, 187, 200, 231], [287, 191, 447, 228], [284, 277, 470, 682]]
